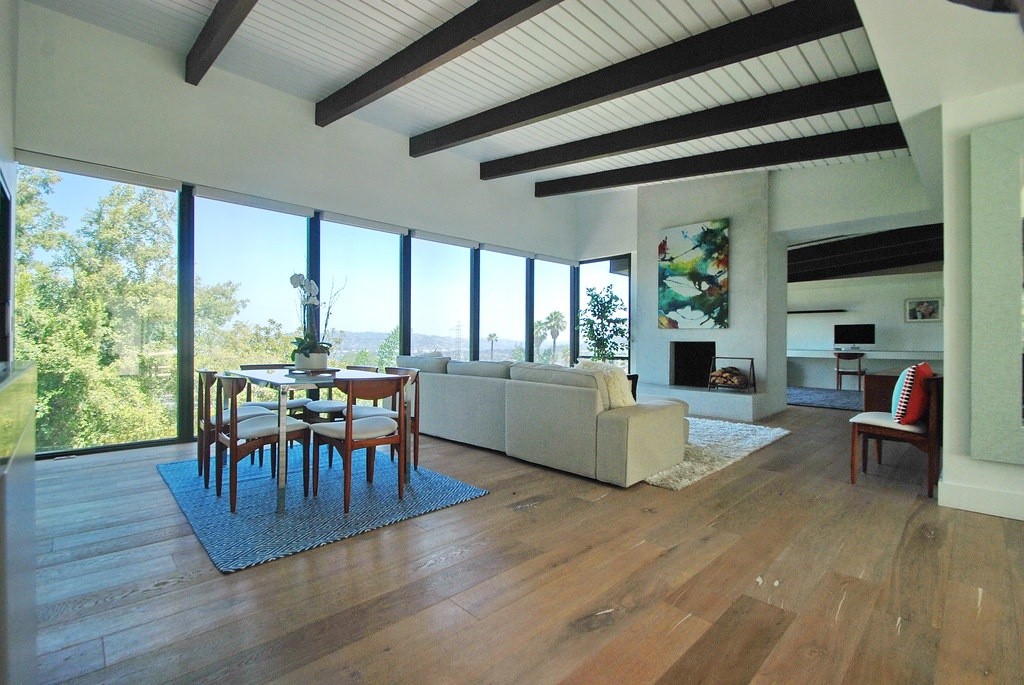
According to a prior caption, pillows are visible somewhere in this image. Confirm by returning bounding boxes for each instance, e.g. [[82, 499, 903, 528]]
[[573, 357, 639, 407], [891, 362, 932, 425]]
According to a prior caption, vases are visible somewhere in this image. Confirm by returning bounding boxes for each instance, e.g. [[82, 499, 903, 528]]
[[296, 353, 327, 368]]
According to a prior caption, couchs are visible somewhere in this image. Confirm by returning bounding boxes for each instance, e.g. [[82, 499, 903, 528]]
[[384, 356, 688, 489]]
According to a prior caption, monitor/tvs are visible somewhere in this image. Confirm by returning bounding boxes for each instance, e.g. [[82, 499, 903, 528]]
[[834, 324, 876, 344]]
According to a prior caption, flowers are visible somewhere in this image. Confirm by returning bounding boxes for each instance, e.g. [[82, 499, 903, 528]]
[[287, 272, 347, 362]]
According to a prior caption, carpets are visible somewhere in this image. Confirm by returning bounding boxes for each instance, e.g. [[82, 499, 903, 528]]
[[156, 444, 489, 573], [645, 417, 792, 492]]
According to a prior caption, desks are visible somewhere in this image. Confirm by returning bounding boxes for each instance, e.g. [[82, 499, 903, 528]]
[[862, 365, 943, 464], [231, 369, 400, 486]]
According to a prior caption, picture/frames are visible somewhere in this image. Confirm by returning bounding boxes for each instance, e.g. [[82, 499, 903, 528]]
[[904, 298, 943, 322]]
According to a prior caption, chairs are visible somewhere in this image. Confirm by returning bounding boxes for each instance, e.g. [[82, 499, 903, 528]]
[[239, 363, 296, 467], [848, 372, 943, 499], [214, 374, 311, 512], [327, 366, 379, 468], [194, 368, 275, 488], [833, 352, 865, 392], [344, 367, 420, 482], [309, 376, 410, 518]]
